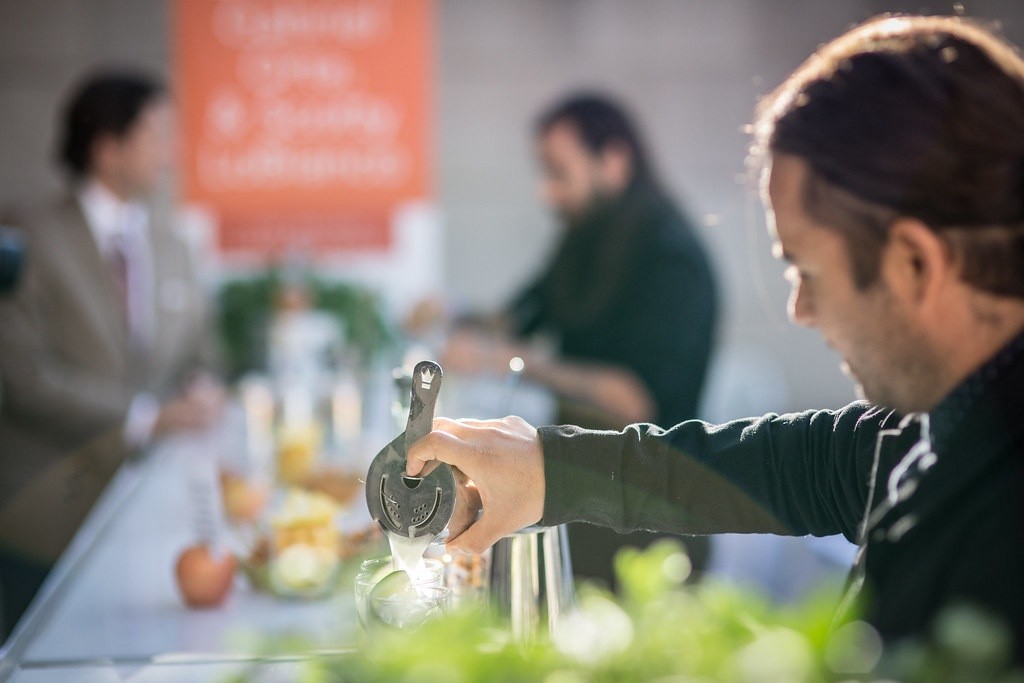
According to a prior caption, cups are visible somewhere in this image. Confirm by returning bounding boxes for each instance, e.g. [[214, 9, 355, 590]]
[[490, 523, 577, 637], [368, 568, 479, 639]]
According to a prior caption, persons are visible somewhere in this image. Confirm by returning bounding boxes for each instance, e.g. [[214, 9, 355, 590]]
[[402, 88, 723, 601], [405, 14, 1024, 683], [0, 64, 227, 649]]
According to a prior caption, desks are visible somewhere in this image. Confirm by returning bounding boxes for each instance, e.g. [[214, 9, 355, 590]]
[[0, 373, 561, 683]]
